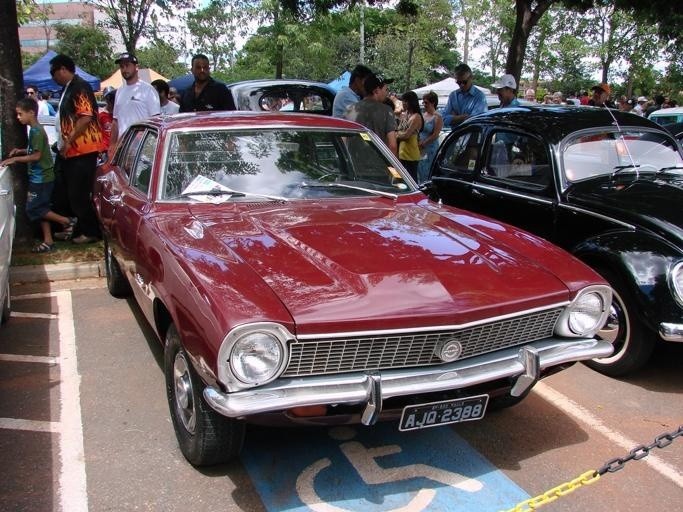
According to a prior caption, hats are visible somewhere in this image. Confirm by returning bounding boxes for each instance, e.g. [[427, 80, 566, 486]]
[[625, 99, 635, 108], [490, 74, 517, 90], [591, 83, 610, 95], [115, 53, 138, 66], [364, 72, 395, 93], [638, 97, 648, 102]]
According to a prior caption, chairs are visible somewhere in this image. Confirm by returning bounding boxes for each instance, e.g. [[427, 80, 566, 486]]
[[467, 141, 503, 171]]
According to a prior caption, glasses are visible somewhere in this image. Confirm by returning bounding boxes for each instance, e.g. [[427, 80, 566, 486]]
[[456, 76, 470, 85], [50, 68, 63, 76], [26, 92, 34, 95]]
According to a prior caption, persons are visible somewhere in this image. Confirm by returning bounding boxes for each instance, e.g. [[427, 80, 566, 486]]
[[526, 85, 677, 121], [1, 48, 236, 254], [327, 63, 520, 188]]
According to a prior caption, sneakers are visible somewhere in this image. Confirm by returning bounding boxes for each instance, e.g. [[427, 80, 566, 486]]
[[30, 241, 56, 253], [64, 216, 78, 240], [73, 234, 100, 244]]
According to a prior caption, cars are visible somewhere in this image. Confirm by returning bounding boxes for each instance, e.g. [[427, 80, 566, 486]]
[[422, 102, 683, 382], [81, 104, 628, 477]]
